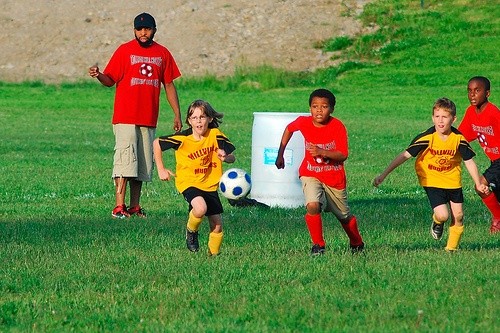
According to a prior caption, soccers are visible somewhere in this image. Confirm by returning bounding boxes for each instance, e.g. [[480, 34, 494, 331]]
[[219, 168, 252, 200]]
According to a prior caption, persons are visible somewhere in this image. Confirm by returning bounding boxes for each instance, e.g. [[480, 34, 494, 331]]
[[373, 97, 491, 253], [89, 13, 183, 218], [153, 100, 236, 257], [457, 76, 500, 232], [275, 88, 365, 257]]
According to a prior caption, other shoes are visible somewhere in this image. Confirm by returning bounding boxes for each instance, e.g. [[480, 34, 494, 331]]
[[490, 221, 500, 234]]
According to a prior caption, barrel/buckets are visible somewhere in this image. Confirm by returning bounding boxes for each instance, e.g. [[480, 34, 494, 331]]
[[250, 112, 325, 209]]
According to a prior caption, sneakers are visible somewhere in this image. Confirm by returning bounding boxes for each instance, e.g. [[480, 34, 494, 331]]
[[112, 204, 130, 219], [128, 205, 147, 218], [311, 244, 325, 256], [208, 251, 222, 257], [186, 227, 199, 252], [431, 221, 445, 240], [350, 242, 364, 255]]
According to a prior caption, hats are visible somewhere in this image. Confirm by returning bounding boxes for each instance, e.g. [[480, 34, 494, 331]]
[[134, 12, 157, 29]]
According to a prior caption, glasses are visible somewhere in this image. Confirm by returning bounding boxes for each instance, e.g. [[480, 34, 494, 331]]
[[190, 116, 209, 121]]
